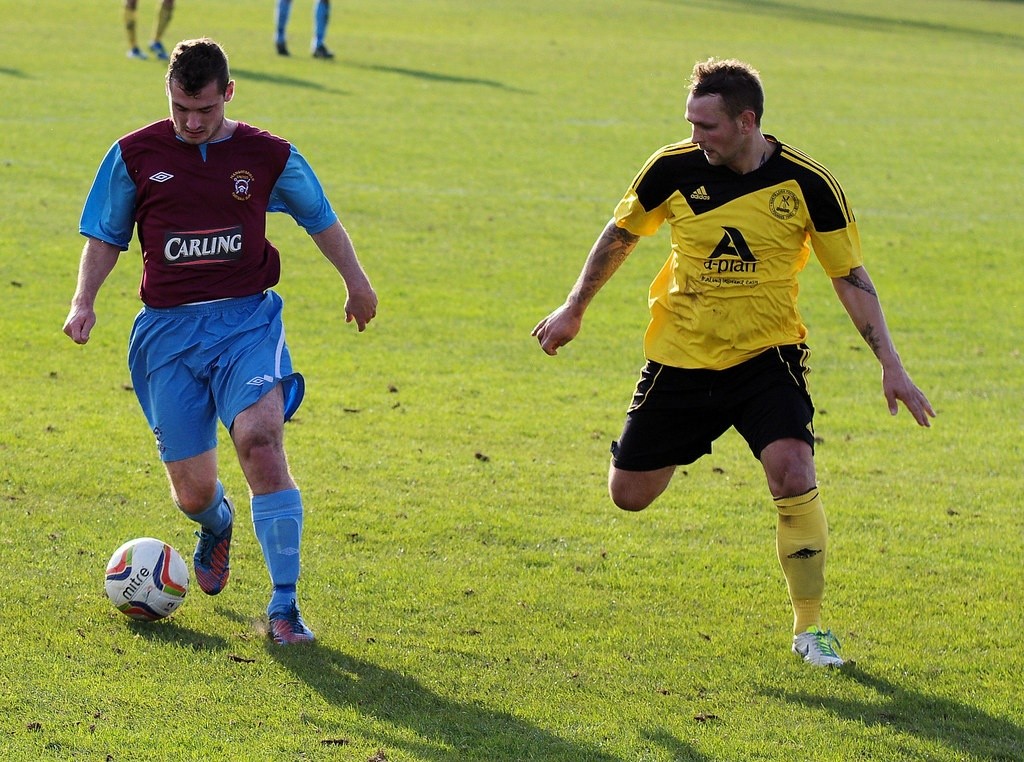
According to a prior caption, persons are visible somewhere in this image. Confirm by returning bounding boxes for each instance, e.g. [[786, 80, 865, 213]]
[[276, 0, 334, 60], [532, 60, 935, 667], [123, 0, 176, 61], [61, 39, 377, 647]]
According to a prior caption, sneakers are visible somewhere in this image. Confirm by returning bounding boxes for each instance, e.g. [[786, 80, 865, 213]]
[[267, 598, 316, 647], [193, 496, 236, 597], [789, 631, 845, 672]]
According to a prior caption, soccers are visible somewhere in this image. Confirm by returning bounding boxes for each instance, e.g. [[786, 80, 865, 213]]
[[103, 537, 189, 623]]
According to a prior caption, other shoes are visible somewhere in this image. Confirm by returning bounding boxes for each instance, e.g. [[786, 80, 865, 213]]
[[126, 47, 150, 61], [310, 44, 334, 60], [274, 36, 289, 54], [147, 42, 168, 61]]
